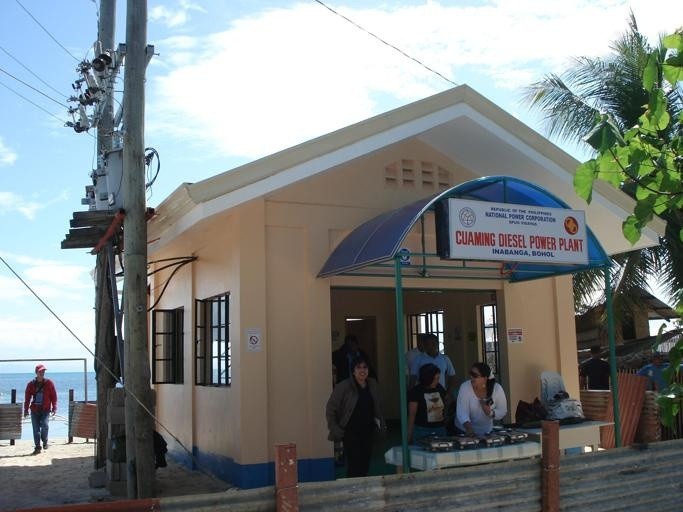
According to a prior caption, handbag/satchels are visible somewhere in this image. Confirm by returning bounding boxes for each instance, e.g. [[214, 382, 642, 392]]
[[515, 398, 547, 421]]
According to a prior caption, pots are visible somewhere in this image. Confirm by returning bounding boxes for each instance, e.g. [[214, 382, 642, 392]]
[[505, 429, 528, 445], [416, 431, 453, 454], [451, 434, 479, 450], [482, 433, 506, 448]]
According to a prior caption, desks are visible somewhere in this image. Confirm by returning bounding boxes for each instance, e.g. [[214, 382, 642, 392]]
[[383, 440, 544, 472], [491, 420, 616, 461]]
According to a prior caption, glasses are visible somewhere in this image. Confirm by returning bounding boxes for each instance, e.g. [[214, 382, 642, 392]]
[[470, 371, 483, 378]]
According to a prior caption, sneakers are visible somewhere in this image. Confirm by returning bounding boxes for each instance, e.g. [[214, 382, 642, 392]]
[[43, 444, 48, 449], [33, 447, 41, 455]]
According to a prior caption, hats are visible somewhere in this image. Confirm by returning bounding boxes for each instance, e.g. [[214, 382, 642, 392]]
[[35, 364, 47, 374], [419, 363, 437, 380]]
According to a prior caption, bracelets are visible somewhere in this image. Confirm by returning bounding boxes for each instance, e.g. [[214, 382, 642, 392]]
[[487, 411, 493, 417]]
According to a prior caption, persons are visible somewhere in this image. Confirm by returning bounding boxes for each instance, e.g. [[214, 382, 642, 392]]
[[325, 358, 388, 476], [406, 334, 425, 371], [454, 362, 507, 437], [580, 345, 611, 391], [410, 333, 457, 402], [636, 352, 683, 388], [332, 335, 374, 386], [406, 363, 451, 445], [24, 365, 58, 455]]
